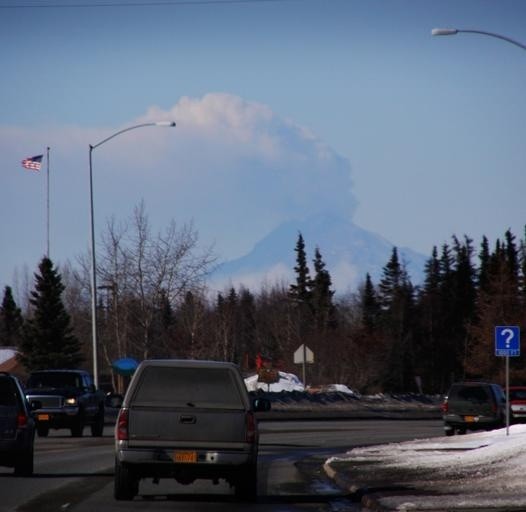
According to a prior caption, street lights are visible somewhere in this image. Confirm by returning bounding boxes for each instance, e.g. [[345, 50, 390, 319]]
[[89, 121, 175, 391], [430, 29, 526, 48]]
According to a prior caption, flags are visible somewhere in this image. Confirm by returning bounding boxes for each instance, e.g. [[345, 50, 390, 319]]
[[19, 154, 42, 171]]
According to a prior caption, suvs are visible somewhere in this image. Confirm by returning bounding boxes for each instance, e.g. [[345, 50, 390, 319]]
[[442, 382, 514, 436], [102, 360, 271, 501]]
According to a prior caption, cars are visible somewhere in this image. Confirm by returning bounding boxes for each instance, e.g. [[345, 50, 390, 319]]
[[24, 367, 105, 436], [503, 386, 526, 422], [0, 371, 34, 475]]
